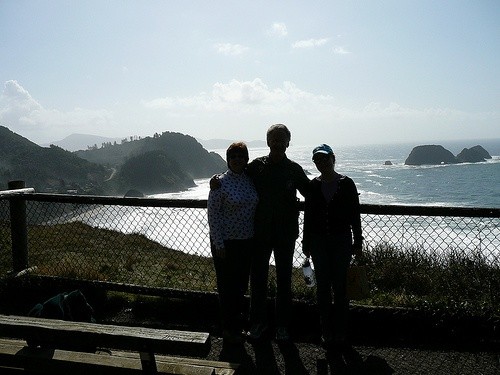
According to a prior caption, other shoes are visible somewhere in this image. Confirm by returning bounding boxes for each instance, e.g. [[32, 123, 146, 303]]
[[275, 325, 289, 340], [250, 324, 268, 338]]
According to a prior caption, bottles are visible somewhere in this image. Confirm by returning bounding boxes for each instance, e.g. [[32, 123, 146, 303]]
[[301, 258, 315, 288]]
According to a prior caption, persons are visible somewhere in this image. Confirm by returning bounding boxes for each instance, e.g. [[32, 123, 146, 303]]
[[303, 145, 363, 343], [207, 143, 299, 345], [211, 127, 361, 342]]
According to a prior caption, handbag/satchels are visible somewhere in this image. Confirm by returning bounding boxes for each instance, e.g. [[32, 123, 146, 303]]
[[346, 259, 368, 299]]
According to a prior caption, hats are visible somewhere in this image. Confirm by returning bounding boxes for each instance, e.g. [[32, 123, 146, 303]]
[[312, 145, 333, 159]]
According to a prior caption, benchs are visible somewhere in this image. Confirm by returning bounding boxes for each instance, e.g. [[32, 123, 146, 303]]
[[0, 314, 212, 375]]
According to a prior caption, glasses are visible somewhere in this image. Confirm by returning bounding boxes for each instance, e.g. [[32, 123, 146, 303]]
[[229, 154, 247, 160]]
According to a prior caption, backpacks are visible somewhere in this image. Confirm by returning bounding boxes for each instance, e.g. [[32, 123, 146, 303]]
[[27, 289, 94, 325]]
